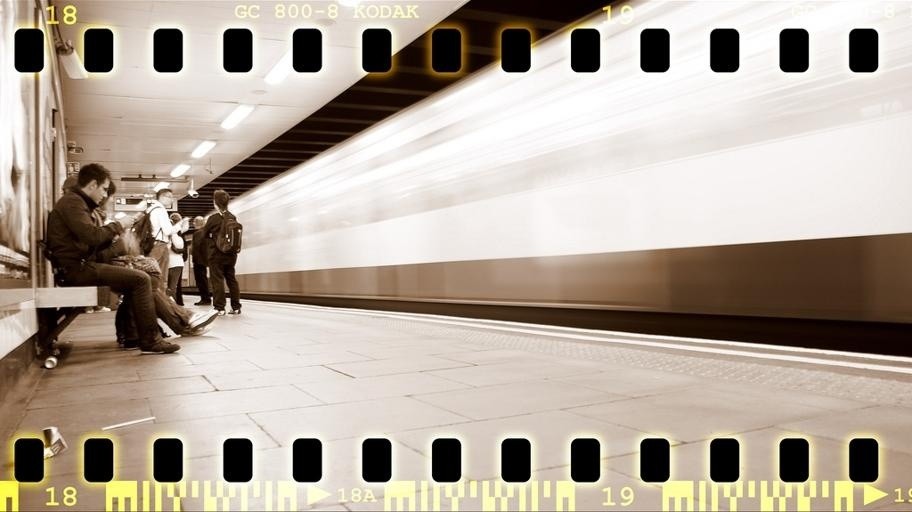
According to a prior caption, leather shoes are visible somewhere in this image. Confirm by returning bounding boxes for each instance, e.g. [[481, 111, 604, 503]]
[[194, 300, 211, 305]]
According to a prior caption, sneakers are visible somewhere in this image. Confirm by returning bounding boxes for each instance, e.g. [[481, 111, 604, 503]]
[[139, 337, 181, 355], [181, 309, 219, 337], [117, 338, 140, 352]]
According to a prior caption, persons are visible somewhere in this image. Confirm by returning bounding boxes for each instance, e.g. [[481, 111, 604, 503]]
[[45, 161, 243, 354]]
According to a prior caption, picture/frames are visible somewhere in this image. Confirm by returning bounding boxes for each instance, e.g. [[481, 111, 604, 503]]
[[52, 109, 68, 209], [0, 0, 40, 307]]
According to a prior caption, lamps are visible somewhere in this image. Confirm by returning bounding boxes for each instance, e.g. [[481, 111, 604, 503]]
[[120, 175, 190, 183], [51, 0, 89, 80]]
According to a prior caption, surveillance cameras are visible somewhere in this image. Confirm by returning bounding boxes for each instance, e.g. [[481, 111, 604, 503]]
[[187, 189, 199, 199]]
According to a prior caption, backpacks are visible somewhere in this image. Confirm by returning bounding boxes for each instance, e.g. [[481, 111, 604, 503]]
[[130, 205, 164, 256], [210, 212, 244, 254]]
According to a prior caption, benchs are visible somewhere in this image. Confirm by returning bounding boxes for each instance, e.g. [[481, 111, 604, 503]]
[[34, 282, 110, 369]]
